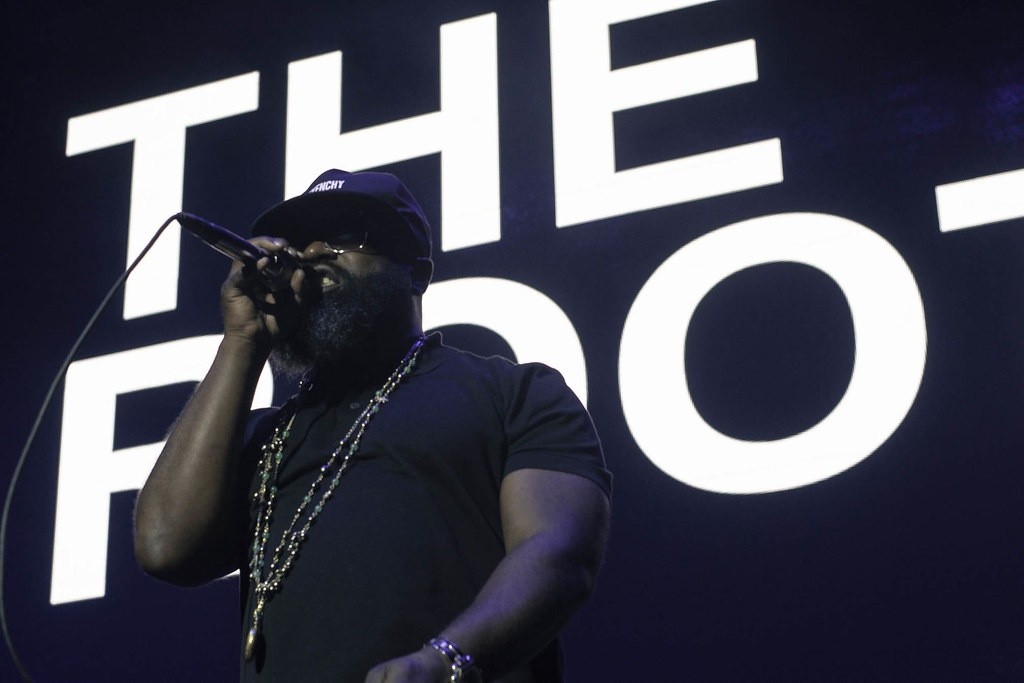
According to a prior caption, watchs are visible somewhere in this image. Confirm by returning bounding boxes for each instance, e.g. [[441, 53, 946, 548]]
[[423, 636, 487, 683]]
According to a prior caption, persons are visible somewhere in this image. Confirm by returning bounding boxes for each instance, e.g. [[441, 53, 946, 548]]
[[131, 169, 614, 683]]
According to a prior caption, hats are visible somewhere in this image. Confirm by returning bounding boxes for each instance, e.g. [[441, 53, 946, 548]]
[[250, 169, 432, 264]]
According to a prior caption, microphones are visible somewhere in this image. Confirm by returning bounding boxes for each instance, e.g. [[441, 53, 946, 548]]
[[176, 211, 301, 296]]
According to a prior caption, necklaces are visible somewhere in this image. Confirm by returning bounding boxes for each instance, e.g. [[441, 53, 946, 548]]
[[244, 335, 427, 663]]
